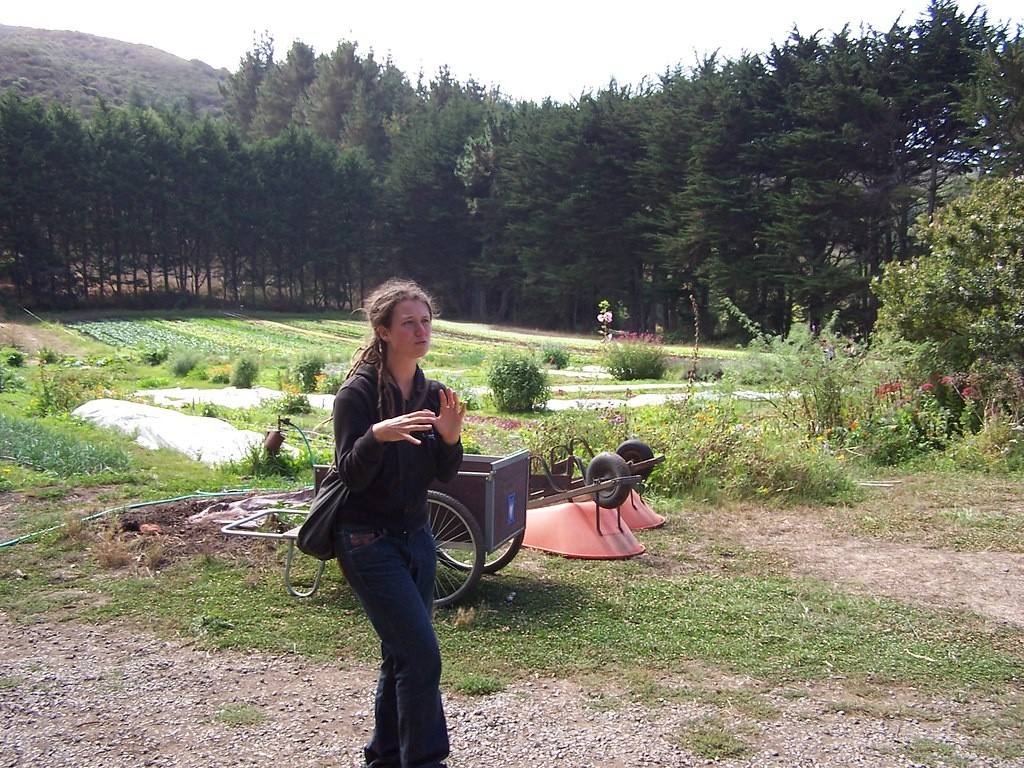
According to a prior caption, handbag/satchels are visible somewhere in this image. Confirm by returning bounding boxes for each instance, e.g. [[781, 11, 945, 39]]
[[297, 461, 355, 560]]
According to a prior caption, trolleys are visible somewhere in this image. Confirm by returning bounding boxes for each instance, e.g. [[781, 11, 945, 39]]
[[220, 414, 533, 610], [508, 436, 667, 560]]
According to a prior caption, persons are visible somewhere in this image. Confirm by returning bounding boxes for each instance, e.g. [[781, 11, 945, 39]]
[[336, 282, 467, 768]]
